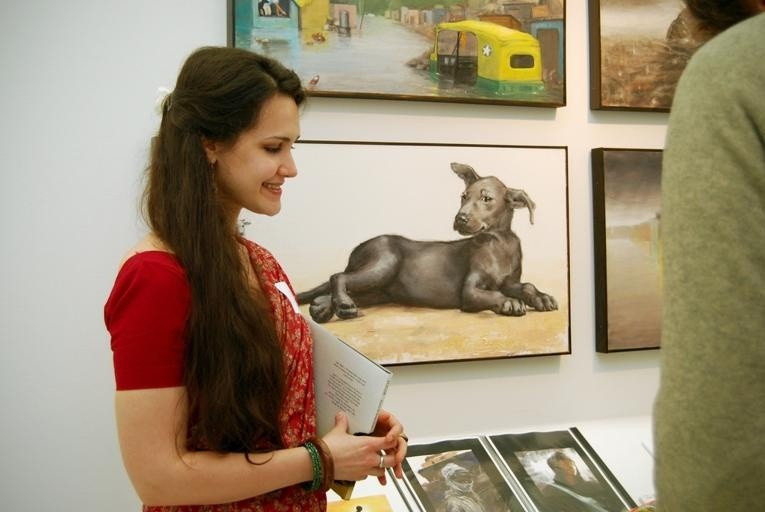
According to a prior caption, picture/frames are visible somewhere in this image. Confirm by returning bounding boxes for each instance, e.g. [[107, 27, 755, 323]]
[[240, 140, 570, 367], [592, 148, 664, 354], [590, 1, 718, 112], [226, 1, 566, 107]]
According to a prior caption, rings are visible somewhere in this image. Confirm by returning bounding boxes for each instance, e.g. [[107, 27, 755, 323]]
[[400, 433, 408, 442], [379, 456, 384, 469]]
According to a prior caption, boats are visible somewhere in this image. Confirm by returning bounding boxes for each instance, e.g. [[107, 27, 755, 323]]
[[310, 75, 319, 85], [312, 33, 326, 42]]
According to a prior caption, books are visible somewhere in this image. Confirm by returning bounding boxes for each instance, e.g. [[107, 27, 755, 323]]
[[306, 315, 393, 500]]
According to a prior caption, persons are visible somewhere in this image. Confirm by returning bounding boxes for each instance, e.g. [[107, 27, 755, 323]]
[[544, 451, 618, 512], [104, 48, 407, 512], [435, 462, 501, 512], [652, 0, 765, 512]]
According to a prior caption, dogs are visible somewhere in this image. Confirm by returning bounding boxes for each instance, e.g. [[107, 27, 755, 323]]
[[291, 162, 558, 324]]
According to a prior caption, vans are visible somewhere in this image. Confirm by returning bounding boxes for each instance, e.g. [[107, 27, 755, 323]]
[[427, 20, 546, 99]]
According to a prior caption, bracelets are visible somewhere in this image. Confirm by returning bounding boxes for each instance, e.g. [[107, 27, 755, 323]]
[[299, 436, 334, 492]]
